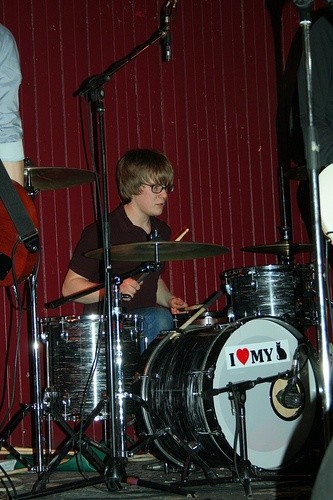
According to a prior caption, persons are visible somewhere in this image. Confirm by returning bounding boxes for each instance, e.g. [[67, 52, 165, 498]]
[[62, 149, 188, 355], [0, 25, 25, 187], [297, 0, 333, 174]]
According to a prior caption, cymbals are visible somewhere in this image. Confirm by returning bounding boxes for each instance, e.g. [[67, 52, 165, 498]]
[[239, 240, 317, 256], [83, 241, 231, 262], [24, 166, 96, 192]]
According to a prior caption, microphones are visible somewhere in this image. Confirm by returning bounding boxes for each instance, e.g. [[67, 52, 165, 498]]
[[159, 0, 171, 61], [285, 348, 300, 396]]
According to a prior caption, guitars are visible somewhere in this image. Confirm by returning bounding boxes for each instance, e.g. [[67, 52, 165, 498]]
[[0, 179, 39, 287]]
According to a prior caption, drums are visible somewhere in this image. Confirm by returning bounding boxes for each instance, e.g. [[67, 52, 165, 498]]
[[173, 309, 228, 331], [39, 312, 144, 423], [137, 315, 327, 477], [219, 261, 317, 327]]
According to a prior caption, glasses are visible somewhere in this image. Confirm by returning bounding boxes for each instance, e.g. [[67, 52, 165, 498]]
[[142, 183, 175, 194]]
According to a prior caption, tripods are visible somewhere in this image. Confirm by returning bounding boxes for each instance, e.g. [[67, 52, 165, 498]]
[[0, 31, 295, 500]]
